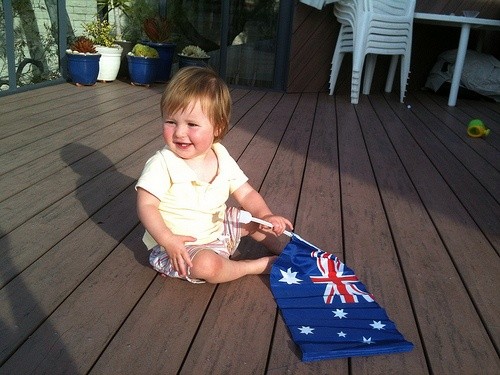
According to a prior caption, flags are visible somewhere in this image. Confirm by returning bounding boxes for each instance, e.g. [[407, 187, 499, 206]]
[[271, 238, 413, 363]]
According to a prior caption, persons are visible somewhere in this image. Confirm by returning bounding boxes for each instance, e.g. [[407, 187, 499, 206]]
[[134, 66, 294, 284]]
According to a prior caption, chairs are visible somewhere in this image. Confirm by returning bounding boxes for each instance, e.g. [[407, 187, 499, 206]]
[[329, 0, 416, 104]]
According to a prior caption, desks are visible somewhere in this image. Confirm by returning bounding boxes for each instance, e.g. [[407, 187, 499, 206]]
[[414, 12, 500, 106]]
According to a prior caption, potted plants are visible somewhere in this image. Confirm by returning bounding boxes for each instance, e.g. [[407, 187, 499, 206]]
[[136, 15, 177, 83], [126, 43, 159, 86], [178, 44, 211, 69], [81, 14, 123, 84], [65, 35, 101, 87], [92, 0, 162, 76]]
